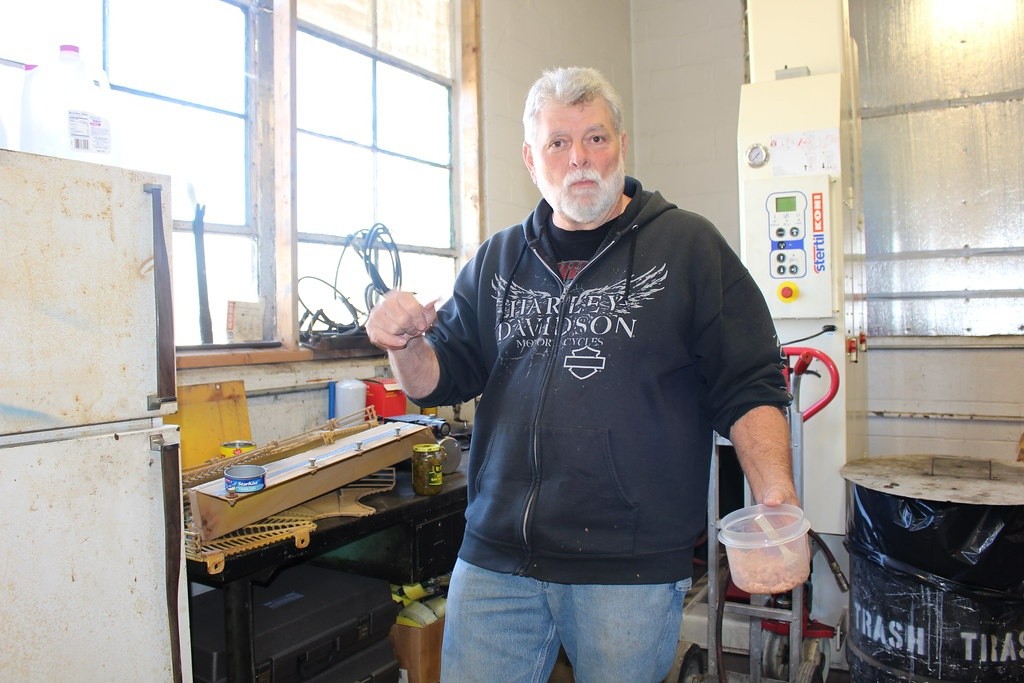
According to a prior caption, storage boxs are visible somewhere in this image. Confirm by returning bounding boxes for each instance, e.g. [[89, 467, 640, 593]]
[[360, 377, 407, 422], [390, 607, 445, 683]]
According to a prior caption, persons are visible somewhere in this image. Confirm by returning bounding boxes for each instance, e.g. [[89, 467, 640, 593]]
[[364, 65, 812, 683]]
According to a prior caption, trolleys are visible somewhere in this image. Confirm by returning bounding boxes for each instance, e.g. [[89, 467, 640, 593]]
[[660, 346, 831, 683]]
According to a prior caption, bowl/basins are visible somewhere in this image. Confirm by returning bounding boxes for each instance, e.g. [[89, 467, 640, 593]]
[[717, 504, 811, 594]]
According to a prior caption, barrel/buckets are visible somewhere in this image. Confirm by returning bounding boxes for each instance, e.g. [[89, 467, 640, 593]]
[[845, 478, 1024, 683]]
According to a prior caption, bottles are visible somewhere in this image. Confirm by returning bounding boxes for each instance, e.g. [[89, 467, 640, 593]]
[[23, 44, 113, 164]]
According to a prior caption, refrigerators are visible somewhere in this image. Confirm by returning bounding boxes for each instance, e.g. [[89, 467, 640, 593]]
[[0, 147, 173, 683]]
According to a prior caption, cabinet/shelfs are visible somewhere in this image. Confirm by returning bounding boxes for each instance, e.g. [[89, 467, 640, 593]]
[[186, 448, 471, 683]]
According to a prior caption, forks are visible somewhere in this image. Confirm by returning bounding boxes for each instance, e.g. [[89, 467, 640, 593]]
[[754, 515, 802, 573]]
